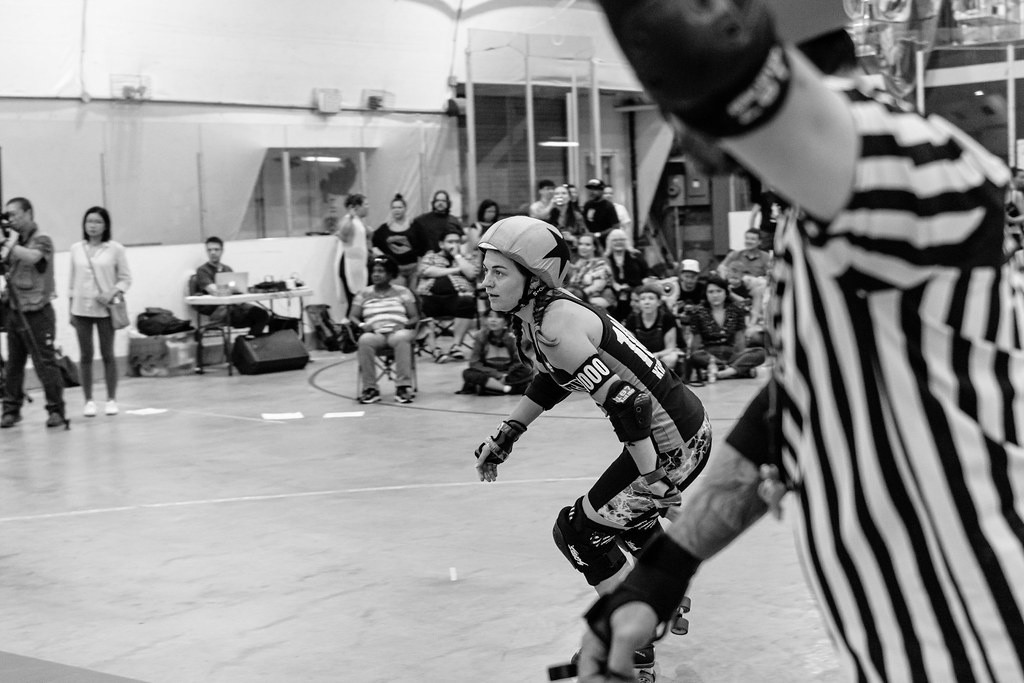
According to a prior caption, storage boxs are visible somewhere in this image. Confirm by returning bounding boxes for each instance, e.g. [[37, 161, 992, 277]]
[[129, 332, 190, 375]]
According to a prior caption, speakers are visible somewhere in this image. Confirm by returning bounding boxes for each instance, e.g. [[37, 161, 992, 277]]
[[231, 328, 310, 376]]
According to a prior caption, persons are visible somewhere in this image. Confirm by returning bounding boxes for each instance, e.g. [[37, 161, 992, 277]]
[[576, 0, 1024, 683], [473, 214, 713, 683], [194, 235, 268, 337], [338, 176, 777, 405], [0, 197, 66, 428], [68, 206, 132, 417]]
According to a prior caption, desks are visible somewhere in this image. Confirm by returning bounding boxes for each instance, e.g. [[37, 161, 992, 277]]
[[185, 288, 314, 377]]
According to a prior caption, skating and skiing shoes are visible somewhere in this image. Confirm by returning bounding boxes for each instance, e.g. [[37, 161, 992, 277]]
[[572, 644, 657, 683]]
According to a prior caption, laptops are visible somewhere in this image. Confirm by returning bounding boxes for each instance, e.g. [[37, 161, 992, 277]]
[[215, 271, 248, 294]]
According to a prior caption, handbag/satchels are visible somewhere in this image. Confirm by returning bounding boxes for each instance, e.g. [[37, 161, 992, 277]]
[[108, 294, 129, 330]]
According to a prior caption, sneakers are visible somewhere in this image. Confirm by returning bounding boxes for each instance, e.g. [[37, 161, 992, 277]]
[[733, 367, 757, 377], [395, 390, 413, 403], [360, 391, 380, 404], [432, 346, 448, 364], [450, 345, 466, 359], [47, 413, 64, 426], [84, 401, 96, 415], [105, 400, 118, 414], [1, 414, 22, 427]]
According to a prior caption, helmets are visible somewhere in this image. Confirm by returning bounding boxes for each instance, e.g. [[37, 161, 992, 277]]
[[476, 216, 569, 290]]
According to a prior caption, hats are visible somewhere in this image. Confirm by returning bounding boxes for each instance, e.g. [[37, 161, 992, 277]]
[[637, 284, 661, 295], [679, 259, 700, 274], [585, 178, 605, 190]]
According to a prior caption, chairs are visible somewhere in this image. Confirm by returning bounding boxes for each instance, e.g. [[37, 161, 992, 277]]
[[355, 281, 485, 395], [191, 275, 235, 377]]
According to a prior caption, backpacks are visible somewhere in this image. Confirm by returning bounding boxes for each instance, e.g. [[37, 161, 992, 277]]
[[137, 306, 194, 336]]
